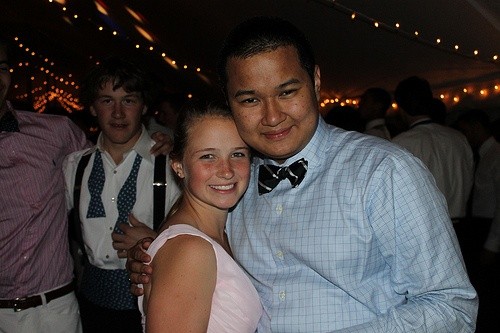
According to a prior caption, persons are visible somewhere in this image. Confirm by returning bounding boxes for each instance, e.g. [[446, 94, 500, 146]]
[[0, 48, 174, 333], [60, 65, 184, 333], [131, 18, 479, 333], [323, 75, 500, 333], [137, 98, 264, 333]]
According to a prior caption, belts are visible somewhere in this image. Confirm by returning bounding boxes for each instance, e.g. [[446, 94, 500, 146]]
[[0, 282, 75, 312]]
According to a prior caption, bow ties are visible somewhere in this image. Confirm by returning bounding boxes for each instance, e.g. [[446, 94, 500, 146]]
[[258, 157, 308, 197], [0, 111, 20, 133]]
